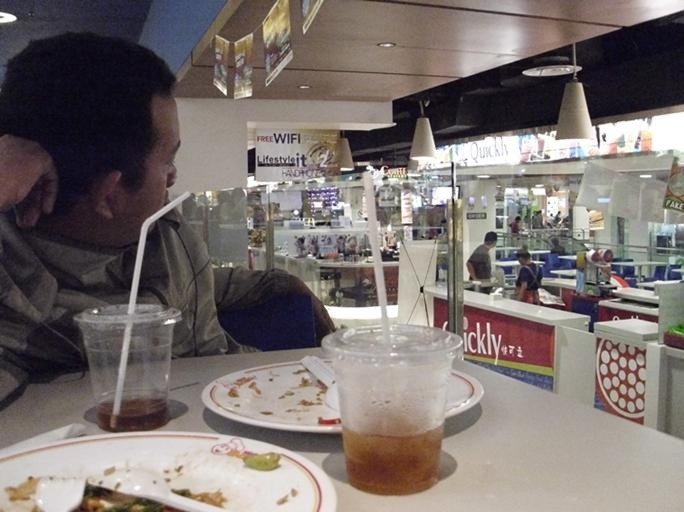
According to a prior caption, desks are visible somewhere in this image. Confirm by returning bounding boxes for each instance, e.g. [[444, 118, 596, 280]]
[[487, 246, 684, 291]]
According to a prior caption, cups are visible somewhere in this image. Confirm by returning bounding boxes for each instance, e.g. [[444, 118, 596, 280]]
[[320, 320, 464, 496], [71, 302, 183, 431]]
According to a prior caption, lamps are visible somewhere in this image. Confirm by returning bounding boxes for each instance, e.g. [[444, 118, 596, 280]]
[[333, 42, 594, 171]]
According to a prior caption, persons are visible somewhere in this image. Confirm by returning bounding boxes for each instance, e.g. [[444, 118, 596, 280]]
[[465, 232, 497, 295], [508, 216, 521, 233], [549, 236, 565, 255], [513, 244, 543, 306], [531, 209, 562, 230], [0, 32, 261, 410]]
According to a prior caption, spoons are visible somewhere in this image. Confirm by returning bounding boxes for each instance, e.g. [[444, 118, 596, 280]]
[[299, 354, 340, 415], [84, 465, 229, 512]]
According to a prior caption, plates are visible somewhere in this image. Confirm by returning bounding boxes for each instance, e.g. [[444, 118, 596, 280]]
[[201, 356, 484, 436], [0, 429, 338, 511]]
